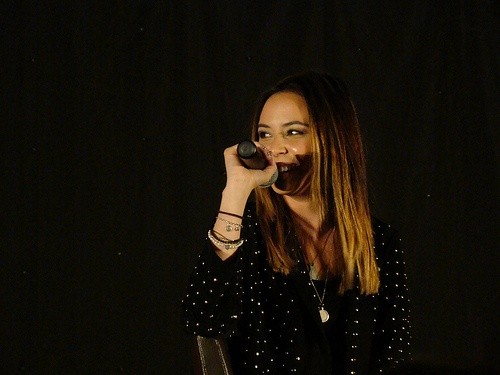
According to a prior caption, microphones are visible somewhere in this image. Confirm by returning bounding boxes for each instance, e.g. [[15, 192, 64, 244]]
[[237, 139, 279, 188]]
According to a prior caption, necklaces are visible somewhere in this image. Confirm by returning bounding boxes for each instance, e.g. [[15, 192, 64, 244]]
[[302, 225, 336, 323]]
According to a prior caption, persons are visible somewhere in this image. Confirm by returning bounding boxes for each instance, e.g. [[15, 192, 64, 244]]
[[180, 72, 416, 375]]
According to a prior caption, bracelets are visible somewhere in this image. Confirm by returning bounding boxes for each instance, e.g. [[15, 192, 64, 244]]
[[208, 230, 243, 252], [215, 217, 243, 231], [217, 211, 243, 219]]
